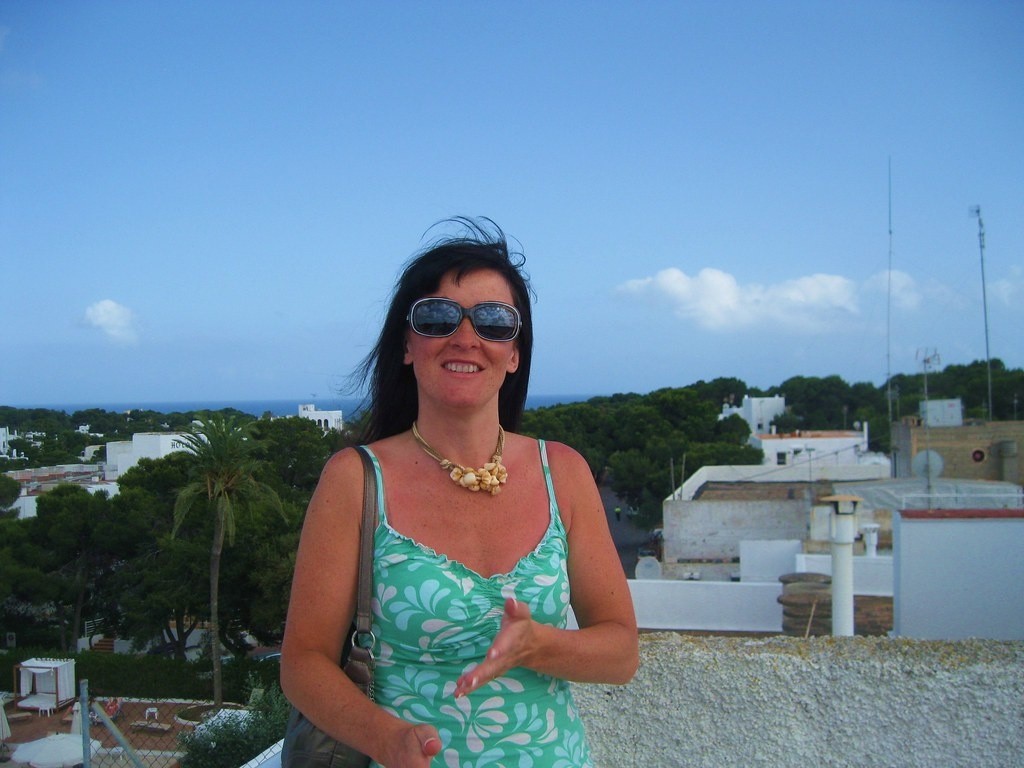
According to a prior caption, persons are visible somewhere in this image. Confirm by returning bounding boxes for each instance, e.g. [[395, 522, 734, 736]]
[[95, 697, 119, 721], [279, 213, 642, 766], [63, 702, 73, 719]]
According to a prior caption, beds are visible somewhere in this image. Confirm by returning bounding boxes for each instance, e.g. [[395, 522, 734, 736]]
[[13, 659, 75, 714]]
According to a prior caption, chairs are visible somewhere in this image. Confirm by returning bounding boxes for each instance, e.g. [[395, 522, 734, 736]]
[[90, 700, 125, 725]]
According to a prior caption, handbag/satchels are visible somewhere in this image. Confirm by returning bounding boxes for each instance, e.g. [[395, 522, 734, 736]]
[[280, 444, 378, 768]]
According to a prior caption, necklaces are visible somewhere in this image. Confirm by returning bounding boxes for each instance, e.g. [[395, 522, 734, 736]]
[[407, 424, 510, 499]]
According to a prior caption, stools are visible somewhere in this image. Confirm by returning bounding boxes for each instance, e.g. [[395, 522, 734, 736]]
[[39, 706, 53, 718], [146, 708, 158, 721]]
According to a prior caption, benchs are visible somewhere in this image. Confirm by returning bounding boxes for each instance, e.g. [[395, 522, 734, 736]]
[[128, 720, 171, 737], [7, 712, 32, 719]]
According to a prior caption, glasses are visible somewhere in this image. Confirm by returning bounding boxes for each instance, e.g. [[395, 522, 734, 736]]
[[406, 297, 523, 342]]
